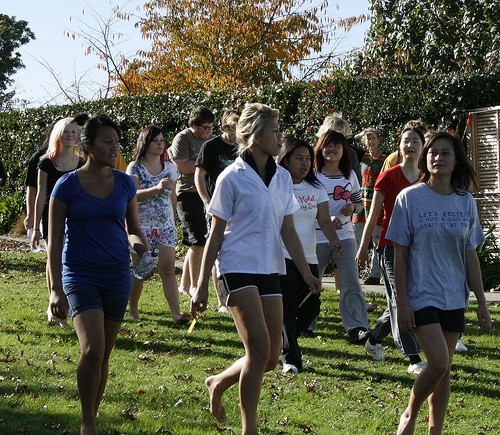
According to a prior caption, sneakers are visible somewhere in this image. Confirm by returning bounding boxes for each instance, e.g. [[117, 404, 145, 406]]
[[407, 360, 428, 375], [282, 362, 298, 374], [363, 337, 386, 362]]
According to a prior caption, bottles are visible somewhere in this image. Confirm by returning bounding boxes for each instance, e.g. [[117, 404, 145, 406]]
[[27, 228, 40, 253], [133, 249, 160, 279]]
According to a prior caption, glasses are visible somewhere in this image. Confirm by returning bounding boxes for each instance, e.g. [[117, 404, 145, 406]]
[[197, 124, 215, 131]]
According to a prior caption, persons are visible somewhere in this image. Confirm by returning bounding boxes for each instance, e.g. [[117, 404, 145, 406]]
[[69, 114, 129, 179], [47, 116, 158, 435], [24, 117, 55, 245], [355, 127, 428, 375], [195, 111, 248, 316], [190, 103, 322, 435], [30, 117, 90, 333], [170, 107, 214, 298], [385, 132, 491, 435], [376, 120, 469, 354], [273, 138, 342, 377], [305, 131, 378, 347], [351, 127, 393, 286], [126, 127, 191, 325], [316, 116, 362, 294]]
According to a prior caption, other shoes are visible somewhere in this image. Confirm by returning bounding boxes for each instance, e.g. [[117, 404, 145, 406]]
[[348, 327, 372, 343], [299, 329, 315, 338], [455, 339, 467, 351]]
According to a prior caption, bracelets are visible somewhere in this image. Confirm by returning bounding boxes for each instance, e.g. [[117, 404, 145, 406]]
[[32, 228, 40, 231]]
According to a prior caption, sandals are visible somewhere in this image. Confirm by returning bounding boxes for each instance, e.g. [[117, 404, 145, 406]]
[[46, 305, 61, 325]]
